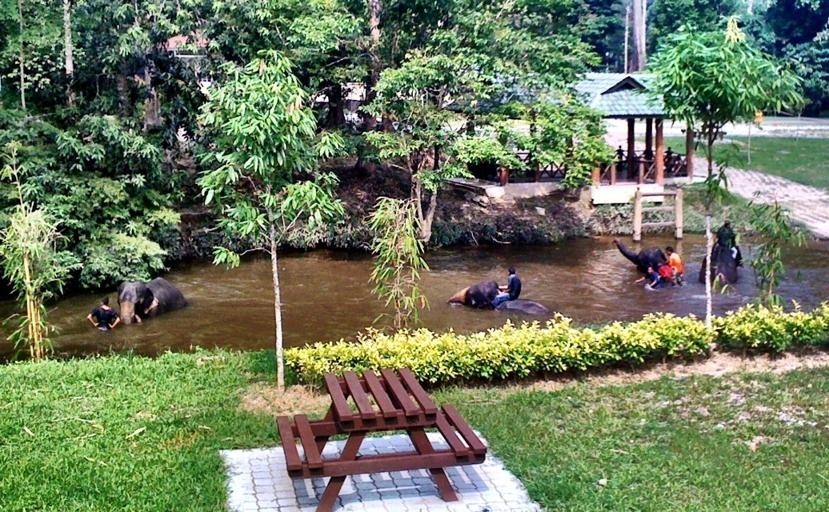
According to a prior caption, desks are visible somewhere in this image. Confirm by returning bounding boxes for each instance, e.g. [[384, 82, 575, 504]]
[[322, 366, 440, 461]]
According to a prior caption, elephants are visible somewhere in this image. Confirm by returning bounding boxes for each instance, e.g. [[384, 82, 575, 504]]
[[699, 246, 738, 286], [116, 276, 188, 326], [446, 280, 550, 318], [612, 237, 686, 287]]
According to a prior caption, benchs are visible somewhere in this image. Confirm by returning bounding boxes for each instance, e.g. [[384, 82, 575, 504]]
[[275, 413, 339, 479], [432, 404, 496, 467]]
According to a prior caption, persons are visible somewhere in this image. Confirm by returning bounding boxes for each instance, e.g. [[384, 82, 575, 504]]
[[87, 296, 121, 331], [635, 246, 684, 288], [490, 266, 521, 306], [716, 220, 743, 266]]
